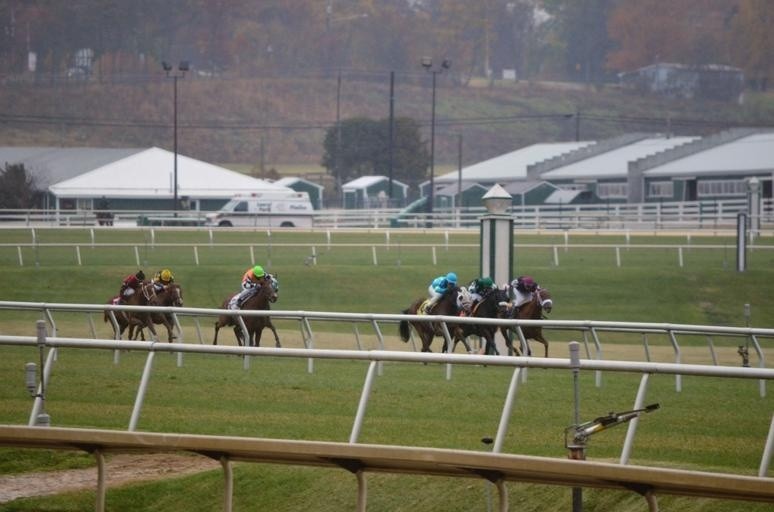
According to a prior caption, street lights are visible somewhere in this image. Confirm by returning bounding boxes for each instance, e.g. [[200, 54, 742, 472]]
[[564, 110, 582, 143], [162, 60, 191, 217], [417, 54, 454, 232]]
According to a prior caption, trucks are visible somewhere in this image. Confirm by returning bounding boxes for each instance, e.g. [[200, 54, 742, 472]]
[[197, 188, 315, 232]]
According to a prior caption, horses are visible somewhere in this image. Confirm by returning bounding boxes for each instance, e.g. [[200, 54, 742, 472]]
[[490, 286, 553, 357], [399, 283, 473, 366], [449, 284, 508, 367], [132, 282, 185, 342], [213, 272, 281, 357], [104, 282, 160, 354]]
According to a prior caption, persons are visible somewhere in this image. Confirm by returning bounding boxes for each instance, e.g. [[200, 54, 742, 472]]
[[421, 271, 457, 314], [232, 265, 271, 310], [465, 277, 497, 304], [147, 269, 174, 295], [506, 275, 538, 315], [113, 269, 146, 305]]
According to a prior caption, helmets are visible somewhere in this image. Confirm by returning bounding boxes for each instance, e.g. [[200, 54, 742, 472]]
[[482, 276, 493, 285], [446, 272, 457, 283], [524, 278, 533, 284], [161, 269, 171, 282], [253, 265, 265, 278]]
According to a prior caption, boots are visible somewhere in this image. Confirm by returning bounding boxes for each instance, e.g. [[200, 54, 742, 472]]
[[425, 306, 430, 313]]
